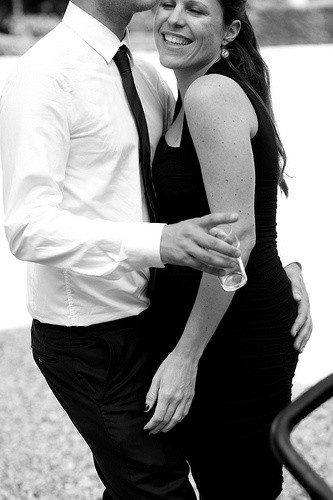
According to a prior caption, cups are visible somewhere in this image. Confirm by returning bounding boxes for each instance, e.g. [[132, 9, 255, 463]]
[[212, 223, 247, 291]]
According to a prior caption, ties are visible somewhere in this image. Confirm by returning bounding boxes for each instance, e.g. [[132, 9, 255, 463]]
[[114, 46, 160, 223]]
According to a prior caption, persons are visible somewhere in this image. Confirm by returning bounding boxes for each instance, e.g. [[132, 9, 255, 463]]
[[1, 0, 314, 500], [138, 0, 304, 499]]
